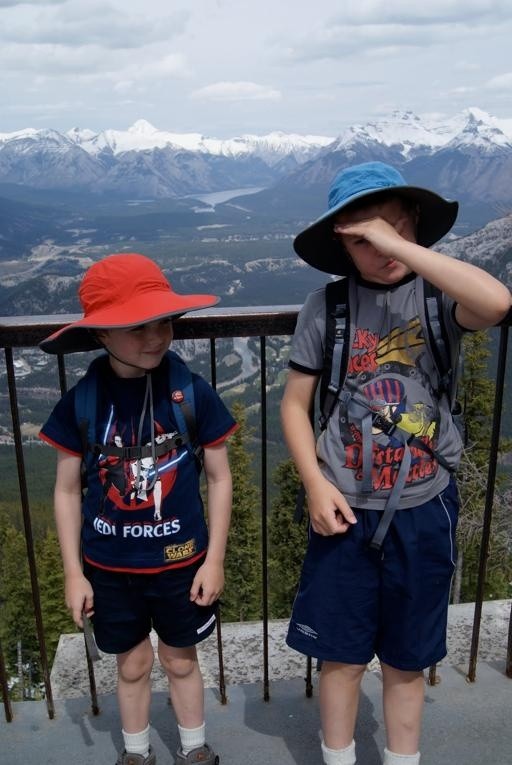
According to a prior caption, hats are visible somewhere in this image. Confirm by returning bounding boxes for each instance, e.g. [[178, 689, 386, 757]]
[[39, 251, 220, 353], [292, 159, 460, 277]]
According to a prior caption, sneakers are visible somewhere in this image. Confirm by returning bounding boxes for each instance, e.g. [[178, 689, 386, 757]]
[[173, 742, 220, 765], [114, 744, 157, 765]]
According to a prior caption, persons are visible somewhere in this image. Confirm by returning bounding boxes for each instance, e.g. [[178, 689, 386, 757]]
[[278, 160, 512, 765], [37, 253, 243, 763]]
